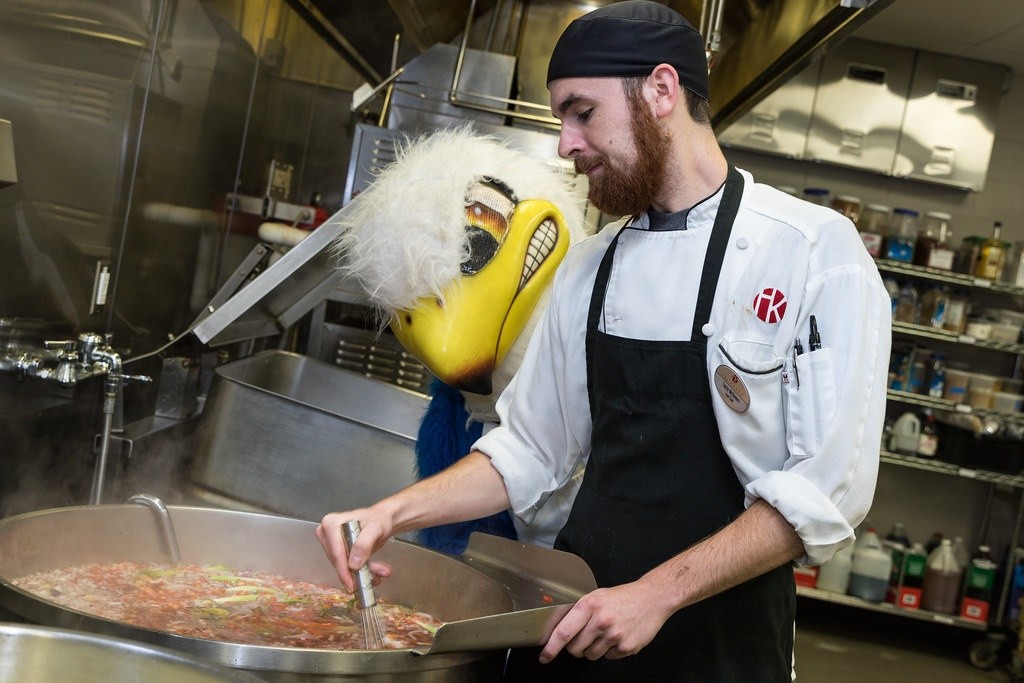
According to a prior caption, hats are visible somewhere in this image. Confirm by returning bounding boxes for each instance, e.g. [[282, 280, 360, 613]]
[[547, 0, 710, 102]]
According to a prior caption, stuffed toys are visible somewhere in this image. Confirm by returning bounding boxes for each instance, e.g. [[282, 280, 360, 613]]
[[344, 134, 588, 552]]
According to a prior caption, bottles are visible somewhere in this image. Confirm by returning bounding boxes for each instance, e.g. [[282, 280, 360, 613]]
[[793, 521, 997, 625], [776, 184, 1024, 341], [880, 341, 1024, 476]]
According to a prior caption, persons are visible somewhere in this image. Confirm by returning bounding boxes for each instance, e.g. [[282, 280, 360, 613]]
[[316, 0, 892, 683]]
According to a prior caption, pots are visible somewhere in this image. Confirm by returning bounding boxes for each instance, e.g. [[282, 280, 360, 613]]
[[1, 501, 516, 683]]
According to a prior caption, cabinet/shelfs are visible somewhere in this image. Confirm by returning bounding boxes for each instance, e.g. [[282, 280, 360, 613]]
[[794, 256, 1024, 668]]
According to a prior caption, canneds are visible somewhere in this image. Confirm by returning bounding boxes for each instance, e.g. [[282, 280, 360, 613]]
[[778, 184, 920, 266]]
